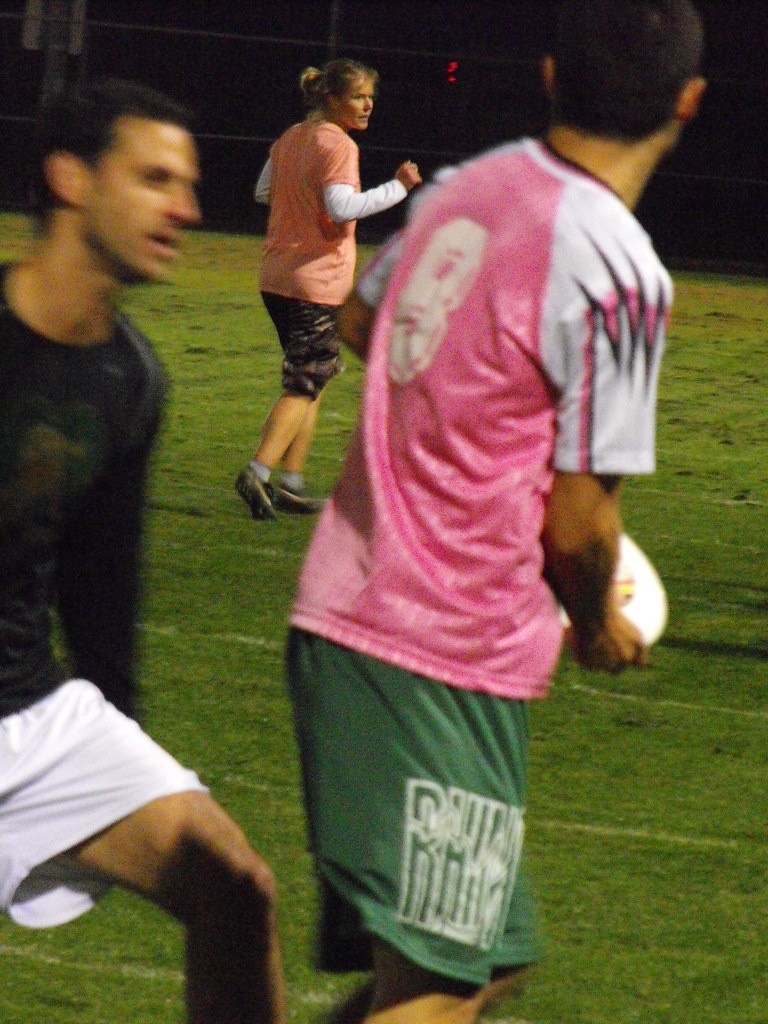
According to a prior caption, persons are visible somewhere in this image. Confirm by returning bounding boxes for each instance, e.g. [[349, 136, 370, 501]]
[[235, 56, 422, 520], [1, 75, 282, 1024], [285, 0, 713, 1024]]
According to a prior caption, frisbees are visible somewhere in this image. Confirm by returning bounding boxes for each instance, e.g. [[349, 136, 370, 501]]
[[561, 529, 669, 649]]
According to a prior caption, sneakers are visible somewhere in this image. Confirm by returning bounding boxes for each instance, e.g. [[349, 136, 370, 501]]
[[270, 473, 323, 513], [235, 466, 280, 526]]
